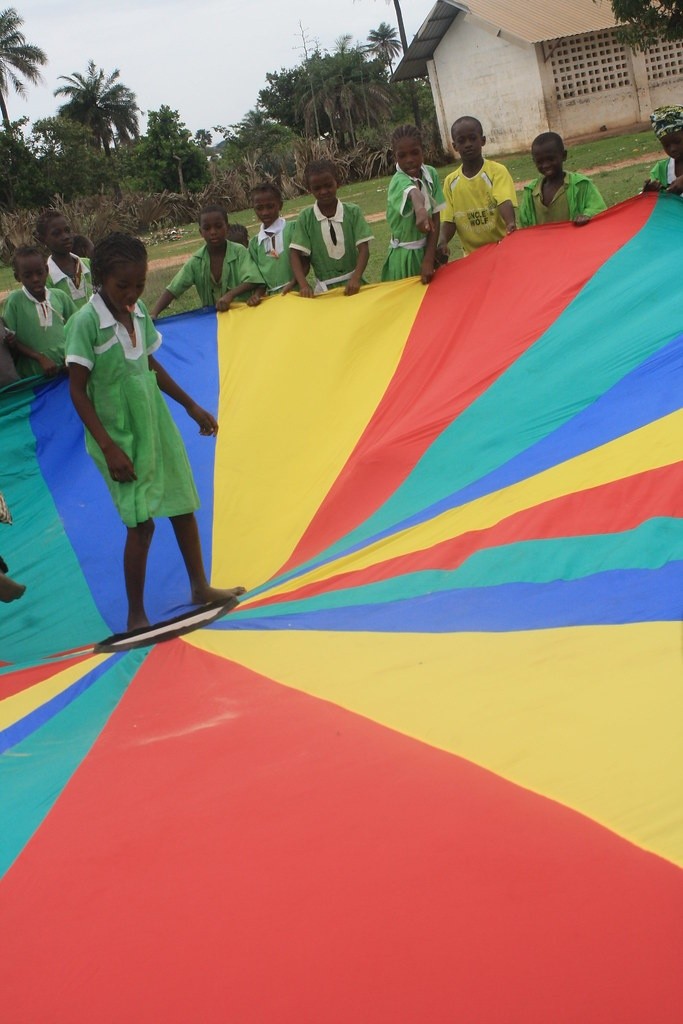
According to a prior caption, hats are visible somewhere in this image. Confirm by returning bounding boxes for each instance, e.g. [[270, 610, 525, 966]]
[[651, 105, 683, 139]]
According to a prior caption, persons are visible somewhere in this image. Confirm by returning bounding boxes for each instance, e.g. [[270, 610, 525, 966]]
[[288, 160, 376, 297], [0, 211, 96, 392], [63, 232, 246, 634], [248, 183, 310, 307], [228, 223, 249, 248], [380, 124, 447, 284], [520, 132, 608, 228], [149, 205, 265, 322], [0, 555, 26, 603], [642, 104, 683, 198], [433, 116, 519, 268]]
